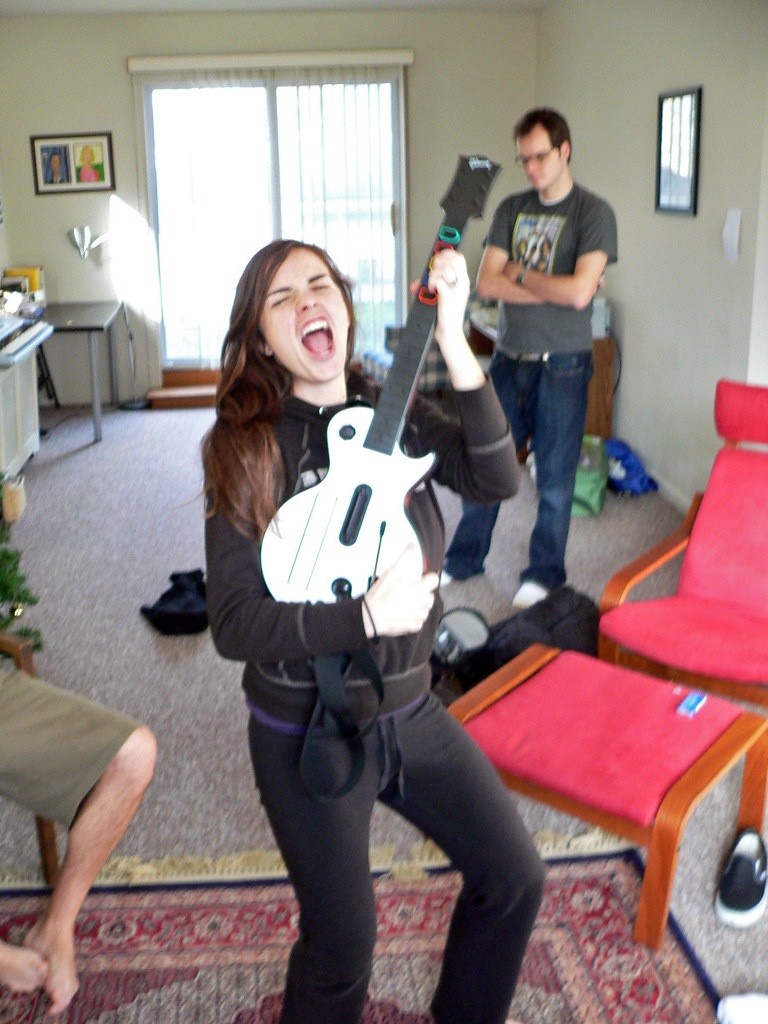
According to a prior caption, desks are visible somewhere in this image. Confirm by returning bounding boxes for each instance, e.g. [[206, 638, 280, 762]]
[[467, 307, 618, 464], [32, 300, 124, 443]]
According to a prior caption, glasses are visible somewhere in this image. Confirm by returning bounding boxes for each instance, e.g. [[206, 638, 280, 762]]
[[515, 143, 557, 165]]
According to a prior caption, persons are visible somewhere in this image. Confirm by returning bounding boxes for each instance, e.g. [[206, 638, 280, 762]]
[[438, 108, 618, 608], [0, 665, 156, 1015], [199, 239, 547, 1024]]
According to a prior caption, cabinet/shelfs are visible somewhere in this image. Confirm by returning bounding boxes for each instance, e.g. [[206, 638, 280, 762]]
[[0, 347, 40, 482]]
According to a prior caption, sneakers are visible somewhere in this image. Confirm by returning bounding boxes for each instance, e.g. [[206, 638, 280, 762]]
[[714, 829, 768, 929]]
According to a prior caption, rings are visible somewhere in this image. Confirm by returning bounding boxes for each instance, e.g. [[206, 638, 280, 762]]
[[447, 279, 458, 287]]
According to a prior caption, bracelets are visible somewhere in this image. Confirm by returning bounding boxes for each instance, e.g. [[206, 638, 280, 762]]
[[517, 267, 528, 286]]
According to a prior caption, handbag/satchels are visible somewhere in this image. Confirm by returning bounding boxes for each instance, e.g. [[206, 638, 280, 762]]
[[432, 586, 600, 696]]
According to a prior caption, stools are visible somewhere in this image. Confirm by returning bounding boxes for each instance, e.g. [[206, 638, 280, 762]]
[[445, 645, 768, 952]]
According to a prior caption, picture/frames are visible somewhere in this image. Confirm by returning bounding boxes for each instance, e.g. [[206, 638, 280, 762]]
[[654, 86, 703, 217], [30, 131, 116, 195]]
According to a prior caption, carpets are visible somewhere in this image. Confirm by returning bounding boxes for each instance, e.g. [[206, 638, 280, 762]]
[[0, 825, 723, 1024]]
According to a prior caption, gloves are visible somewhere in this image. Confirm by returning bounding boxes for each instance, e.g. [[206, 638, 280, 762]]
[[364, 550, 439, 639]]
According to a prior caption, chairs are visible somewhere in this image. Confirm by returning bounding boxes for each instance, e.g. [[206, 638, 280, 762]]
[[599, 378, 768, 710]]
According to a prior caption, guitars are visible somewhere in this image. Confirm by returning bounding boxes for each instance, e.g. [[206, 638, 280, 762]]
[[261, 152, 503, 604]]
